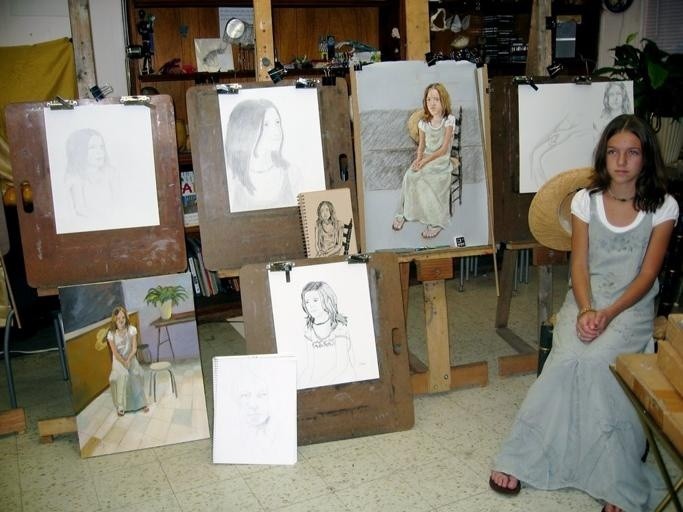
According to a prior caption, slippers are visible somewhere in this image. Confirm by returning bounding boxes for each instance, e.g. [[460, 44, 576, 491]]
[[118, 410, 124, 416], [489, 472, 521, 494], [422, 225, 443, 238], [601, 504, 623, 512], [392, 217, 405, 230], [144, 406, 149, 412]]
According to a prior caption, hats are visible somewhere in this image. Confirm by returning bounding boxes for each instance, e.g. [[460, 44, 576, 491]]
[[408, 109, 428, 144], [95, 328, 109, 351], [528, 169, 597, 252]]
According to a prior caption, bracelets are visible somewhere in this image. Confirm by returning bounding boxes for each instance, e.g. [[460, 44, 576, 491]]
[[577, 305, 596, 321]]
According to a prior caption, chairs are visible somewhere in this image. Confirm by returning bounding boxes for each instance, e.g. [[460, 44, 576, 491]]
[[539, 180, 683, 463]]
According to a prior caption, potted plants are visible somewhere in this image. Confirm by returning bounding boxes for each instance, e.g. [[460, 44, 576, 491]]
[[593, 31, 683, 167]]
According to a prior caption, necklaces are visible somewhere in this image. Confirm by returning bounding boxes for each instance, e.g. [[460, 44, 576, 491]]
[[607, 187, 636, 203], [310, 318, 339, 340]]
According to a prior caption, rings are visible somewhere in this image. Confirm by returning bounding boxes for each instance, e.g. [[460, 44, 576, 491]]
[[577, 332, 582, 338]]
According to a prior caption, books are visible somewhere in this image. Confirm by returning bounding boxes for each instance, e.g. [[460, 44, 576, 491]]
[[183, 238, 242, 299]]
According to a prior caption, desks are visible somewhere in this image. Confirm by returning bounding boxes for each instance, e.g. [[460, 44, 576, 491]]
[[607, 355, 683, 512]]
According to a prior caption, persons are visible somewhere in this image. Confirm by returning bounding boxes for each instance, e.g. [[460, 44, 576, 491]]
[[600, 82, 631, 122], [490, 117, 679, 512], [300, 281, 351, 350], [136, 10, 155, 74], [224, 99, 302, 212], [107, 307, 150, 416], [314, 201, 344, 258], [393, 83, 456, 238]]
